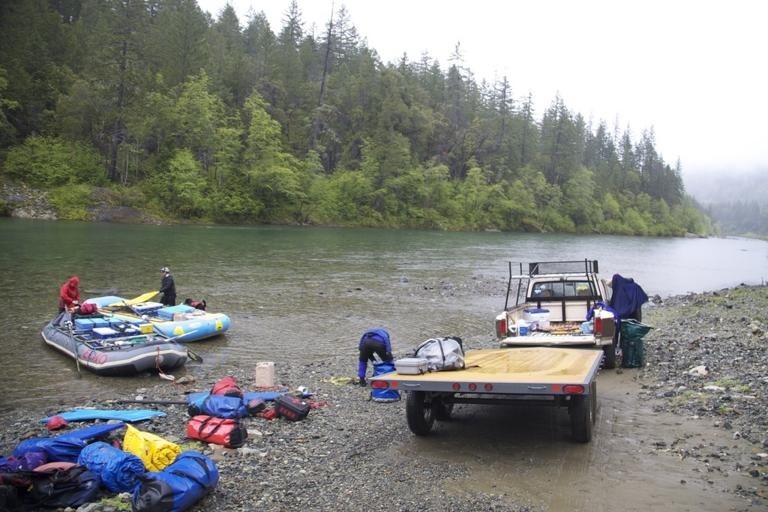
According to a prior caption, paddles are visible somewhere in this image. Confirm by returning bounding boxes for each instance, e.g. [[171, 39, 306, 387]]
[[125, 330, 197, 352], [63, 301, 82, 379], [122, 300, 204, 363]]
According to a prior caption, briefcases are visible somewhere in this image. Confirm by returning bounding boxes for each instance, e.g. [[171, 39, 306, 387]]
[[274, 392, 311, 422]]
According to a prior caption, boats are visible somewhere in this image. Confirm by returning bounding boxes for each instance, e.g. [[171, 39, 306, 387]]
[[41, 296, 231, 375]]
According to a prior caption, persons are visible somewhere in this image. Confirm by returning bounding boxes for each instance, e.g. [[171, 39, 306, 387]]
[[160, 268, 176, 306], [610, 274, 649, 355], [358, 327, 395, 387], [57, 276, 80, 314]]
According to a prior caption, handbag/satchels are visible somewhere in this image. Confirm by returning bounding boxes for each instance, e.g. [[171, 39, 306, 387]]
[[133, 377, 250, 512], [370, 334, 466, 403]]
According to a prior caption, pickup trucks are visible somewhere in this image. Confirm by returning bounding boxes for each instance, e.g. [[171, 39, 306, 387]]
[[494, 258, 619, 369]]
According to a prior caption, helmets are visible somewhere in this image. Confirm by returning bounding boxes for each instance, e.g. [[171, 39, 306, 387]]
[[159, 267, 170, 273], [47, 415, 68, 431]]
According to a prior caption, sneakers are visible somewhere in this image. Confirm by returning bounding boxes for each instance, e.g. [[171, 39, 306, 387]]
[[359, 380, 366, 386]]
